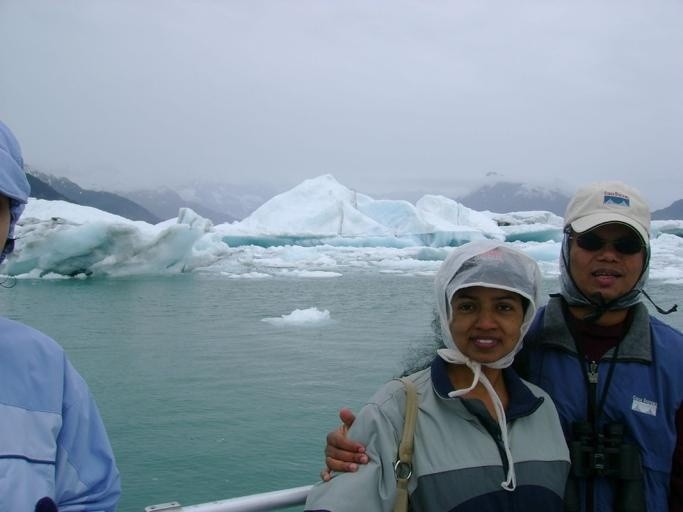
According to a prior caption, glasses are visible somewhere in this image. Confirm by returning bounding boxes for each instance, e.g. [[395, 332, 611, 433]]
[[568, 230, 642, 254]]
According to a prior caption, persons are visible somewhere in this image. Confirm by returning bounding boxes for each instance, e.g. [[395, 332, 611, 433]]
[[317, 179, 683, 512], [299, 238, 575, 511], [0, 117, 125, 511]]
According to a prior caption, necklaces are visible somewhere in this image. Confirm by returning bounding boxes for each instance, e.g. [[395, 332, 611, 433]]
[[452, 363, 501, 394]]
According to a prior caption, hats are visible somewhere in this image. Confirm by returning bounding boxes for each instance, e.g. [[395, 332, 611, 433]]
[[562, 180, 651, 248], [0, 120, 32, 203]]
